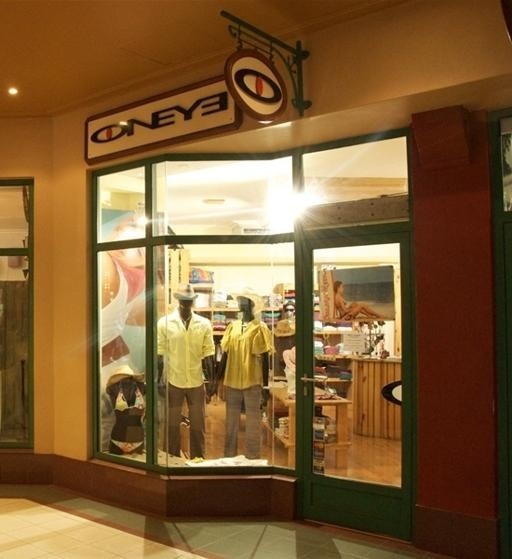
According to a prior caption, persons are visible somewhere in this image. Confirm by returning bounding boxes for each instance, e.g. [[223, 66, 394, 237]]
[[283, 300, 296, 320], [98, 213, 164, 454], [105, 365, 147, 456], [209, 291, 278, 458], [333, 279, 387, 321], [154, 292, 217, 461]]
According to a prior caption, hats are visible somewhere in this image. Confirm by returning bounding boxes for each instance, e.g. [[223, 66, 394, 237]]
[[272, 318, 296, 339], [231, 284, 263, 312], [173, 283, 199, 302], [104, 365, 146, 394]]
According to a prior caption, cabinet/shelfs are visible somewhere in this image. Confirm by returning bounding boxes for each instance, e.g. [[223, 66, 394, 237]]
[[100, 208, 190, 452], [260, 381, 352, 469], [191, 286, 285, 403], [311, 328, 369, 398]]
